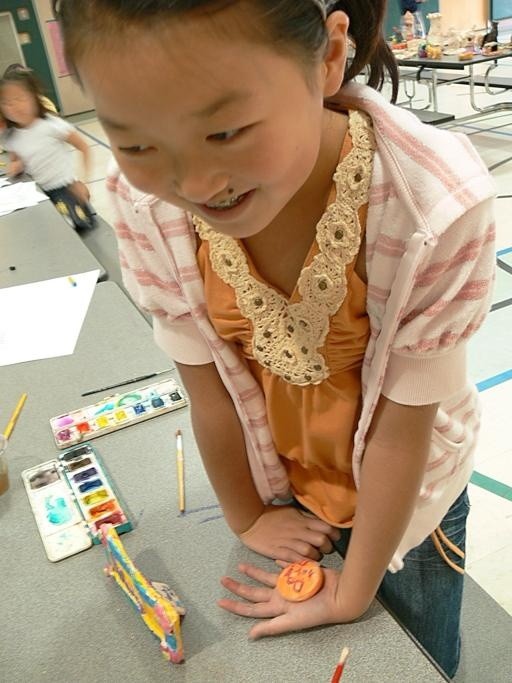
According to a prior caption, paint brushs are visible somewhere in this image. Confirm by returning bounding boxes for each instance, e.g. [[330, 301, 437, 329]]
[[82, 367, 176, 396], [331, 646, 351, 683]]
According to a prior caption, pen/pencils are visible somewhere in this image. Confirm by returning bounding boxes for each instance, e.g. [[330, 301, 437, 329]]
[[176, 429, 185, 511], [3, 392, 28, 439]]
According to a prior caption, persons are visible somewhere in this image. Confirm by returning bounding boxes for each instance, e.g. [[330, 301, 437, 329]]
[[48, 0, 498, 677], [0, 63, 95, 233]]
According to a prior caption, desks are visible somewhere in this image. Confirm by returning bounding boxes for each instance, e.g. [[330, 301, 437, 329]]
[[346, 50, 512, 113], [0, 281, 454, 682], [0, 145, 108, 288]]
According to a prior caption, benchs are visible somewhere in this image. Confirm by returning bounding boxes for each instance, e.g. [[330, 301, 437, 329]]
[[401, 105, 454, 125], [346, 62, 512, 89]]
